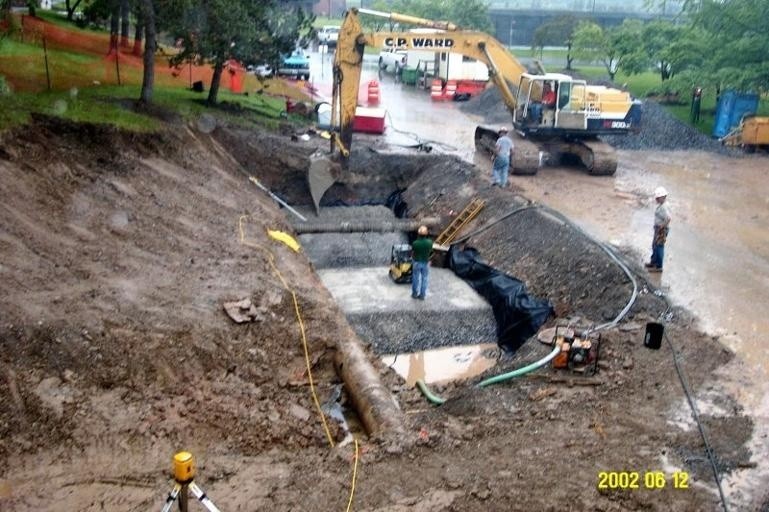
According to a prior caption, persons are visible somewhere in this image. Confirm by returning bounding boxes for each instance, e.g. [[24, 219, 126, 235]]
[[645, 187, 670, 273], [411, 226, 433, 299], [690, 89, 702, 121], [531, 83, 557, 122], [492, 127, 514, 189]]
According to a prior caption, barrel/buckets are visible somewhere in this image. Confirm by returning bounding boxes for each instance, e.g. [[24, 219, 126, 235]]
[[368, 81, 379, 104], [644, 322, 664, 350], [446, 82, 456, 96], [432, 79, 442, 100]]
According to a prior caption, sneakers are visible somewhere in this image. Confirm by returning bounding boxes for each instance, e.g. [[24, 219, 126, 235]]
[[645, 261, 655, 267], [648, 268, 663, 272]]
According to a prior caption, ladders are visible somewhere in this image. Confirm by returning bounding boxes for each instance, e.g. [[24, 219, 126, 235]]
[[429, 196, 486, 259]]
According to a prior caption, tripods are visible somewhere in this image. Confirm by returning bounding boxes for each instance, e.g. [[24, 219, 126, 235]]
[[160, 482, 220, 512]]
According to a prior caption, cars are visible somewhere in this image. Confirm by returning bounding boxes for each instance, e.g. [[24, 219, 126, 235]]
[[277, 48, 310, 81]]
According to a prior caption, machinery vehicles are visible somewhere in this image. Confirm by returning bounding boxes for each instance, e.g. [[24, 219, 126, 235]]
[[298, 5, 650, 219]]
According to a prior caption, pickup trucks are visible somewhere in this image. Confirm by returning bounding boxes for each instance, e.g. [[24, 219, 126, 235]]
[[315, 24, 340, 47], [377, 47, 407, 76]]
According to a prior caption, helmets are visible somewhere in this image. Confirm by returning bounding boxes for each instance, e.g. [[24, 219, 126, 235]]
[[499, 127, 508, 133], [655, 186, 668, 199], [418, 225, 429, 235]]
